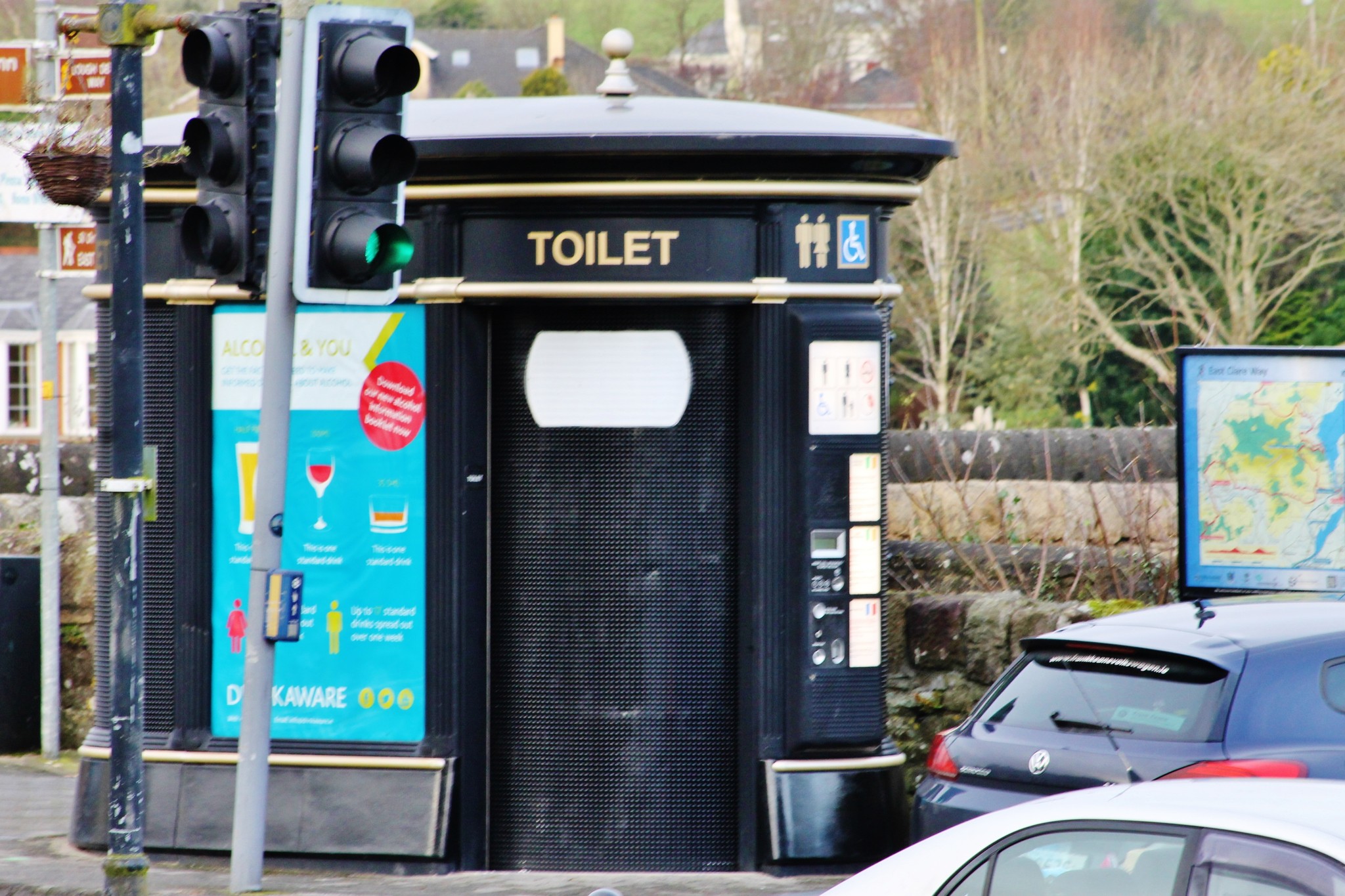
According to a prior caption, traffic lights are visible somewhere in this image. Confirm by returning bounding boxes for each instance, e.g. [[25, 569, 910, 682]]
[[177, 0, 283, 299], [293, 4, 420, 306]]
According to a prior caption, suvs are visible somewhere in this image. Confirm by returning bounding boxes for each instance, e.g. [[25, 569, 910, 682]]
[[913, 590, 1344, 839]]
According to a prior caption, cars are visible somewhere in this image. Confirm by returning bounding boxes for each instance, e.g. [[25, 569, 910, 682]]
[[819, 778, 1345, 896]]
